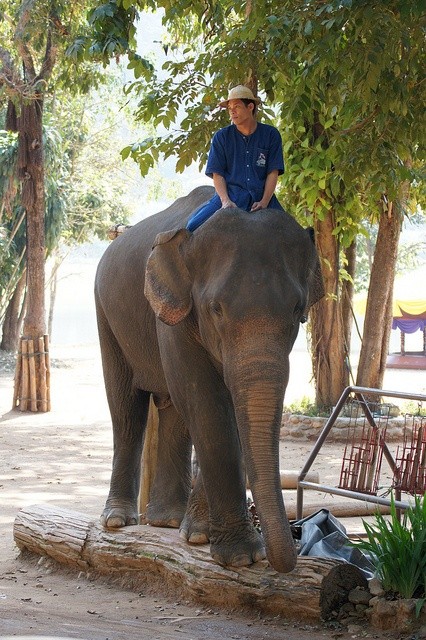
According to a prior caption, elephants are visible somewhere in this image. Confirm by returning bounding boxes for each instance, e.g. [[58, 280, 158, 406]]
[[94, 184, 327, 574]]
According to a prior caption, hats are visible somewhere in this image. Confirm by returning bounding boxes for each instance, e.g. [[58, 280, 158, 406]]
[[218, 85, 261, 107]]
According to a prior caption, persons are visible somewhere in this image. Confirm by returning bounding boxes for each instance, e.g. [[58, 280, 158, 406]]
[[186, 86, 285, 234]]
